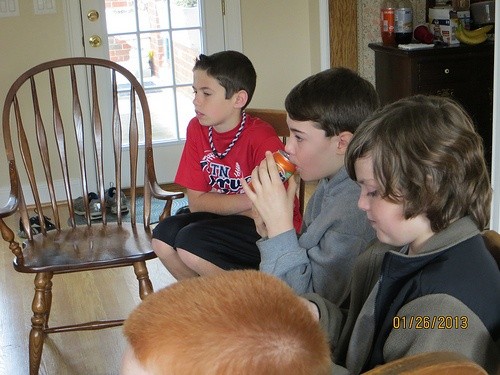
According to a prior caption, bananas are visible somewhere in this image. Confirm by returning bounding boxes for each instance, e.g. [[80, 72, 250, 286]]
[[453, 17, 494, 45]]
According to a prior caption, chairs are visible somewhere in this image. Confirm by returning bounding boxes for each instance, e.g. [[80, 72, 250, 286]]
[[0, 58, 185, 372], [245, 109, 306, 219]]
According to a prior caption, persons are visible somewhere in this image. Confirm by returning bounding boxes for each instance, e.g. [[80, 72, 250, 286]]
[[149, 49, 303, 293], [294, 95, 500, 375], [240, 67, 405, 362]]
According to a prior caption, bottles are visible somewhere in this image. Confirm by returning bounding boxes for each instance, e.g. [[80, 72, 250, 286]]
[[380, 0, 397, 43], [394, 0, 413, 45]]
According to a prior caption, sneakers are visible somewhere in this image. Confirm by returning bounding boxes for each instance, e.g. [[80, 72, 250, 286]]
[[72, 192, 102, 220], [104, 187, 129, 214], [20, 215, 56, 238]]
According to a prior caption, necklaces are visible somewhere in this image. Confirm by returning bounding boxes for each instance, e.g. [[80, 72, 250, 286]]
[[208, 110, 246, 159]]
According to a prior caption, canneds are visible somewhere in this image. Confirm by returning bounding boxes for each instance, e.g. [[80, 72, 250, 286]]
[[247, 150, 297, 192]]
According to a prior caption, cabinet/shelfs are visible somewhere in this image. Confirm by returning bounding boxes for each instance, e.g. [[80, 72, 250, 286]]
[[368, 42, 495, 185]]
